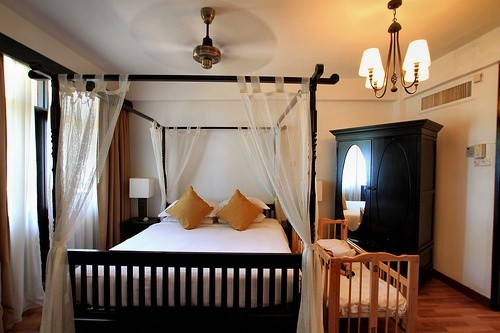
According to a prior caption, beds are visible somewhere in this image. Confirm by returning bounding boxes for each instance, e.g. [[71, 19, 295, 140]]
[[26, 63, 339, 333], [291, 218, 419, 333]]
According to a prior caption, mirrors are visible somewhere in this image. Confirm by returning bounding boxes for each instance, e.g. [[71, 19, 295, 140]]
[[341, 144, 368, 230]]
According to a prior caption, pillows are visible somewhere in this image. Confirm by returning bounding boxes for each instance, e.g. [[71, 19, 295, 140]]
[[158, 185, 270, 231]]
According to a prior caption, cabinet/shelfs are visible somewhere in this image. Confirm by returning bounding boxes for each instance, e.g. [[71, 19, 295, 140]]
[[330, 118, 445, 290]]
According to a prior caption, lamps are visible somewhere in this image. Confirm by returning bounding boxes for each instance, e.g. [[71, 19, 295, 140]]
[[359, 0, 431, 98], [128, 177, 154, 222]]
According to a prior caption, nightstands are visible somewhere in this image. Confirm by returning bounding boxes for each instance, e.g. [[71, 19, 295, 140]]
[[281, 220, 293, 250], [119, 216, 162, 243]]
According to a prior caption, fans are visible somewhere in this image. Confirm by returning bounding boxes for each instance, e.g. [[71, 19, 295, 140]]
[[132, 0, 279, 77]]
